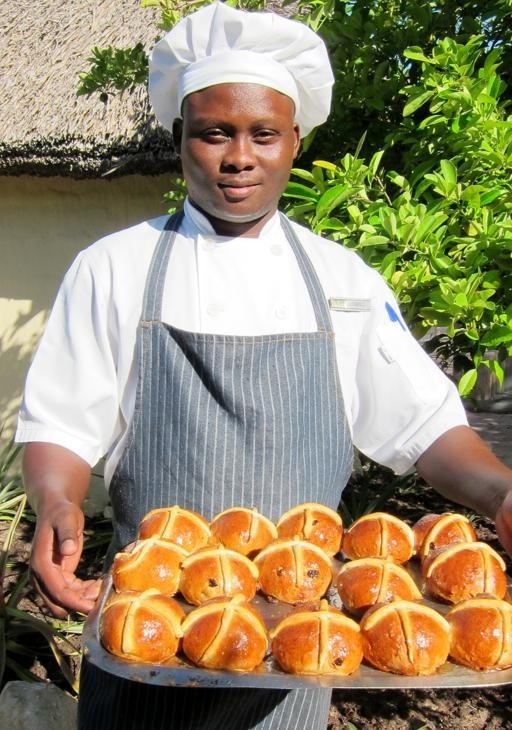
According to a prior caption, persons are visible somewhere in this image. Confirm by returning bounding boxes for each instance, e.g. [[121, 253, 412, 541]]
[[9, 0, 511, 728]]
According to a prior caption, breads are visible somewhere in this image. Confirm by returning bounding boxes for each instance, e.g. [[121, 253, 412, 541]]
[[210, 508, 278, 562], [137, 505, 210, 553], [180, 594, 268, 674], [443, 593, 511, 672], [412, 512, 477, 573], [113, 536, 188, 597], [253, 540, 333, 606], [337, 555, 423, 617], [276, 503, 343, 558], [178, 543, 259, 606], [425, 541, 507, 605], [360, 600, 452, 676], [341, 511, 414, 566], [102, 588, 187, 663], [268, 599, 364, 675]]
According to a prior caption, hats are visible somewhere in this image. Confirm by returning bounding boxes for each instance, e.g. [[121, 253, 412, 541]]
[[149, 1, 334, 139]]
